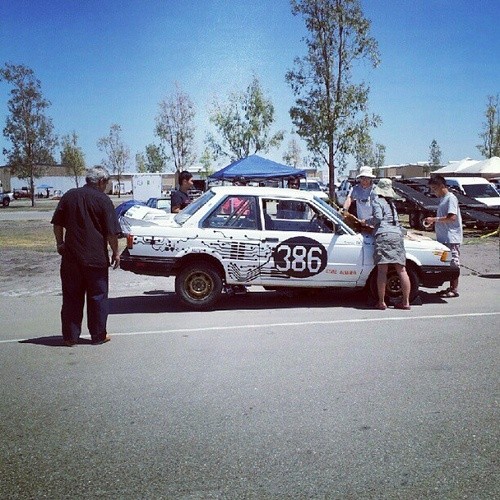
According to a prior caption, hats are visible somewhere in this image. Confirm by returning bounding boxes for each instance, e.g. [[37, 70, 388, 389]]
[[370, 178, 400, 199], [355, 166, 377, 180]]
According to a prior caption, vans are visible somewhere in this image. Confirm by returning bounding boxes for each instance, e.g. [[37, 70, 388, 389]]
[[443, 176, 499, 212]]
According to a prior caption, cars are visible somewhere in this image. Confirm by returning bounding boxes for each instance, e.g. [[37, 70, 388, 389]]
[[335, 179, 353, 207], [278, 179, 330, 204], [118, 184, 461, 310], [0, 186, 30, 206]]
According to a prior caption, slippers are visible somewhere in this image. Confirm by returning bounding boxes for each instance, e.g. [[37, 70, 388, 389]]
[[394, 304, 411, 309], [375, 303, 388, 309], [436, 288, 455, 294], [440, 290, 460, 298]]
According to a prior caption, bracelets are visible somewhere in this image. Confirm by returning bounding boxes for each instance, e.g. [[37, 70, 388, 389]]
[[57, 241, 63, 245], [437, 217, 440, 223]]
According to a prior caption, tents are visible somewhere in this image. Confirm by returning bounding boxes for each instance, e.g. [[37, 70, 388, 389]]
[[430, 157, 480, 177], [457, 155, 500, 179], [36, 184, 53, 198], [208, 154, 308, 190]]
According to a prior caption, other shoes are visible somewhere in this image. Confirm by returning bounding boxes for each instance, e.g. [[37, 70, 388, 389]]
[[66, 339, 78, 346], [94, 337, 112, 344]]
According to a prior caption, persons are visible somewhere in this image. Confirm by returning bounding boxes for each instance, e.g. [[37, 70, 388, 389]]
[[39, 188, 50, 198], [423, 176, 462, 298], [342, 166, 378, 220], [275, 175, 309, 220], [222, 176, 251, 216], [171, 171, 193, 213], [49, 165, 120, 348], [361, 178, 411, 310]]
